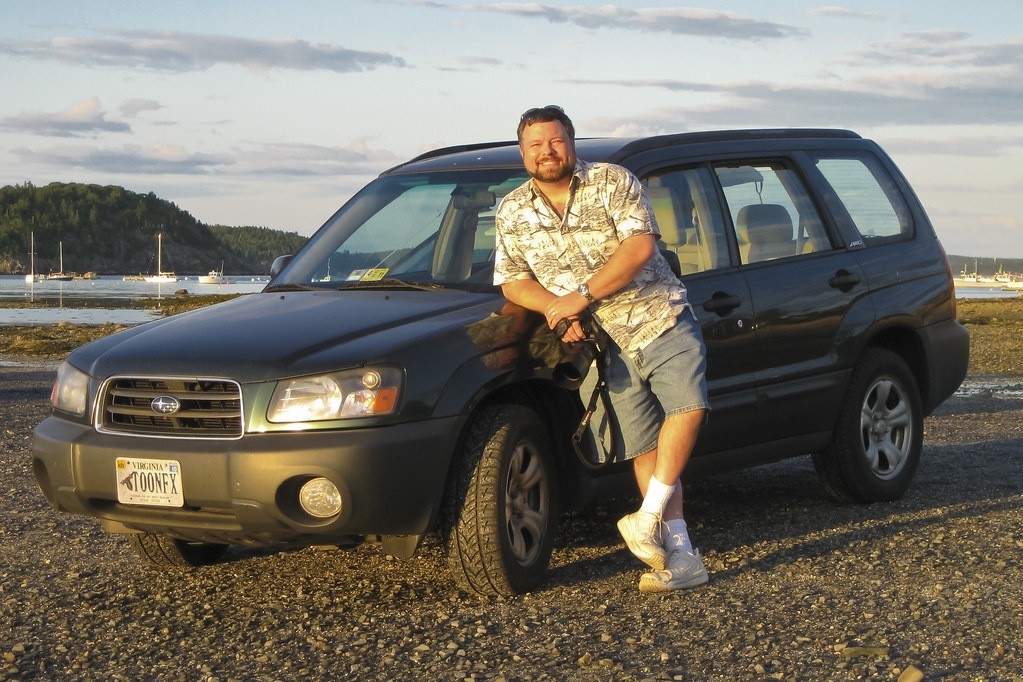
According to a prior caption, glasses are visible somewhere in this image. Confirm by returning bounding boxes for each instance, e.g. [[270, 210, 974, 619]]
[[520, 105, 564, 121]]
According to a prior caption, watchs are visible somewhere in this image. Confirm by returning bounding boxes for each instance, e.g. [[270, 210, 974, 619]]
[[577, 282, 597, 305]]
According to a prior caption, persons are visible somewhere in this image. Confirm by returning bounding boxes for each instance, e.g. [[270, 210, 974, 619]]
[[491, 104, 712, 591]]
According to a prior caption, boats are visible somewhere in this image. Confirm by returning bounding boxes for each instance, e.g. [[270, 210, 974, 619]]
[[199, 264, 236, 284], [1006, 276, 1023, 287], [82, 271, 97, 280], [994, 269, 1023, 282], [159, 272, 179, 281], [961, 264, 982, 282]]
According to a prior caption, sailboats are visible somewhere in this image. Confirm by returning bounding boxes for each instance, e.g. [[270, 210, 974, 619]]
[[26, 232, 42, 283], [144, 233, 177, 283], [311, 257, 333, 282], [44, 241, 73, 281]]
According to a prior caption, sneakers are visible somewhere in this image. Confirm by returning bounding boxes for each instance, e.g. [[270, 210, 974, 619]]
[[640, 547, 709, 591], [617, 510, 670, 570]]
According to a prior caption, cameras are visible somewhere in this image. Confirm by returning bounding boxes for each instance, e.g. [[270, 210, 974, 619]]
[[552, 331, 604, 390]]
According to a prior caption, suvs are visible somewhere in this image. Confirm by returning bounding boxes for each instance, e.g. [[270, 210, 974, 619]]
[[30, 130, 969, 601]]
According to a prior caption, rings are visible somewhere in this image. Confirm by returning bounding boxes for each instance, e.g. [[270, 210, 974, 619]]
[[552, 311, 557, 319]]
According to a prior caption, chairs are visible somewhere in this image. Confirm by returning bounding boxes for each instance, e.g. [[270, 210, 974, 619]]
[[646, 186, 687, 254], [737, 203, 793, 265]]
